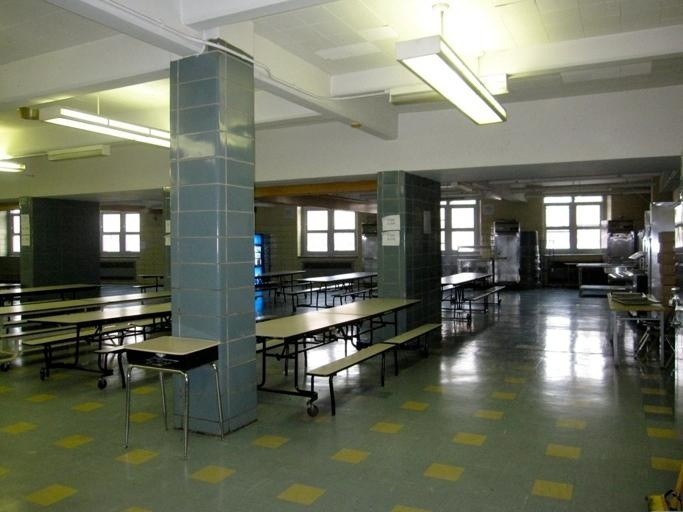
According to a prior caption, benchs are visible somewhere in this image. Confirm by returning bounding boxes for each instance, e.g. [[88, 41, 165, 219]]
[[284, 286, 334, 309], [467, 287, 506, 328], [307, 323, 443, 419], [331, 286, 378, 305], [22, 325, 147, 387]]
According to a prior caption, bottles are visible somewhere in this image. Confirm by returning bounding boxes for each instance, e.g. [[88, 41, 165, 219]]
[[255, 277, 262, 285]]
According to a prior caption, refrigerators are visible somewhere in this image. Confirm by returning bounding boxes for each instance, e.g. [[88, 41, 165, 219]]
[[254, 233, 270, 274]]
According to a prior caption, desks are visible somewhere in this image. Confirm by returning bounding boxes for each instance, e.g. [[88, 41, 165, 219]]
[[1, 279, 170, 313], [5, 306, 171, 390], [121, 334, 224, 457], [607, 293, 668, 370], [296, 267, 378, 309], [438, 270, 495, 319], [244, 297, 422, 417], [254, 267, 306, 304]]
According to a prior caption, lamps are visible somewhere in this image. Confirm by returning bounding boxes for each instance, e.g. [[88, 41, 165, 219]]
[[384, 4, 509, 126], [32, 96, 171, 149]]
